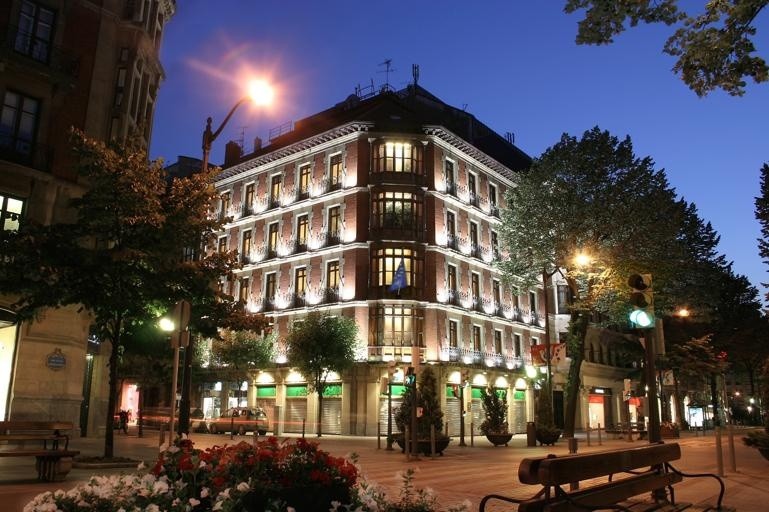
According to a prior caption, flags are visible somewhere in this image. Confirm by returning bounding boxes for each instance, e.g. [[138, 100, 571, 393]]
[[390, 260, 407, 293]]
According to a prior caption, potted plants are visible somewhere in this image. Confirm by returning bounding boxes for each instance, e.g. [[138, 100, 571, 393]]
[[393, 367, 450, 455], [533, 382, 561, 444], [476, 385, 513, 445]]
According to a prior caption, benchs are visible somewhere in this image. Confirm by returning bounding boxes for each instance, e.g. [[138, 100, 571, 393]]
[[0, 420, 81, 456], [475, 442, 727, 512]]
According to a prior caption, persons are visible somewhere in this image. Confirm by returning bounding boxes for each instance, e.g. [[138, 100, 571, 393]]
[[115, 410, 129, 434]]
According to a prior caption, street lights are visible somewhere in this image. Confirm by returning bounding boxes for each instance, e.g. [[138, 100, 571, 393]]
[[177, 78, 276, 438], [543, 255, 593, 426]]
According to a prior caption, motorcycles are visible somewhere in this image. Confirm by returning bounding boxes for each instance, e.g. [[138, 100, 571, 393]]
[[119, 411, 129, 435]]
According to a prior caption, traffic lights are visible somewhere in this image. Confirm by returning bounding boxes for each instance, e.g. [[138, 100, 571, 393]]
[[628, 273, 655, 329]]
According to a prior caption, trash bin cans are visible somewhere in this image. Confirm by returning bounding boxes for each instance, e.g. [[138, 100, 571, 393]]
[[526, 422, 536, 446]]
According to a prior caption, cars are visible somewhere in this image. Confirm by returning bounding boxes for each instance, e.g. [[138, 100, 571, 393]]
[[208, 407, 269, 436]]
